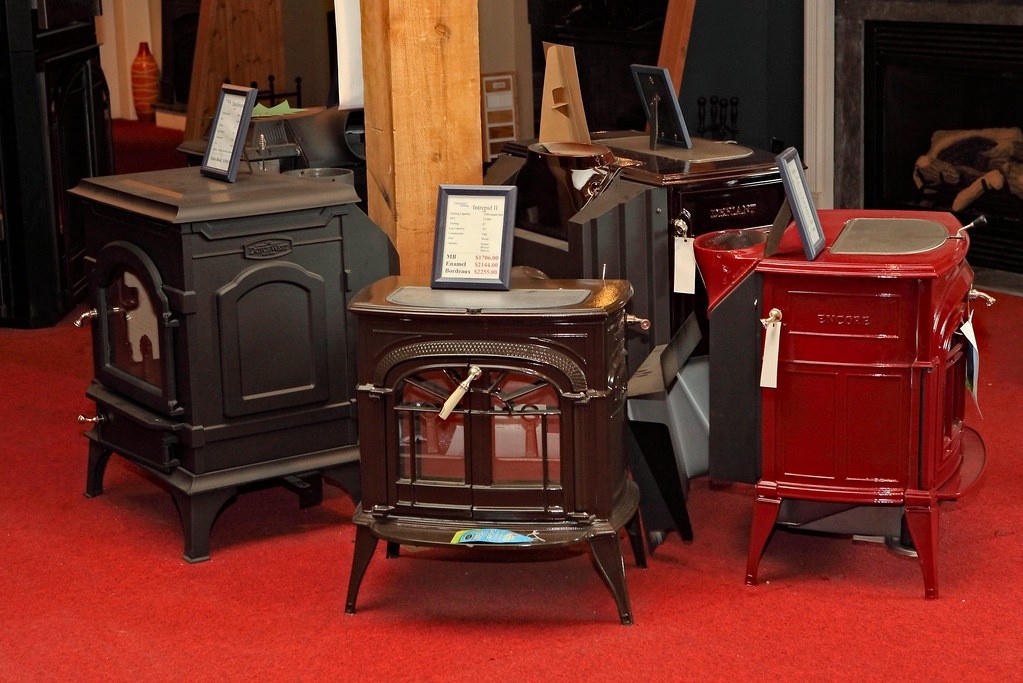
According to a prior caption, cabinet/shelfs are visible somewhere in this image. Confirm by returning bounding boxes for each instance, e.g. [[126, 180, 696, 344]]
[[0, 0, 112, 332], [342, 272, 639, 626], [743, 210, 988, 600], [578, 131, 810, 361], [81, 166, 364, 563]]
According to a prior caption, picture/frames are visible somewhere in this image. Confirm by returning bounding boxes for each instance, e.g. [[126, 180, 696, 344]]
[[199, 84, 258, 183], [632, 64, 692, 149], [764, 146, 825, 260], [429, 184, 518, 287]]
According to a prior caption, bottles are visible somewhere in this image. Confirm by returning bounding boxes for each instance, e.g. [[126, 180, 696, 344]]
[[131, 42, 162, 123]]
